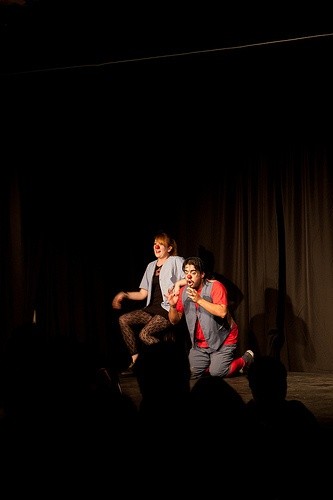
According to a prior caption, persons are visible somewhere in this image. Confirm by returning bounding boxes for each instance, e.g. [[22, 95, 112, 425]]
[[162, 256, 253, 377], [113, 232, 192, 375], [2, 325, 333, 500]]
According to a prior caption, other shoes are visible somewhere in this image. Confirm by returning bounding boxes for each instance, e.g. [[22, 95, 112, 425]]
[[241, 350, 254, 376], [117, 362, 143, 376]]
